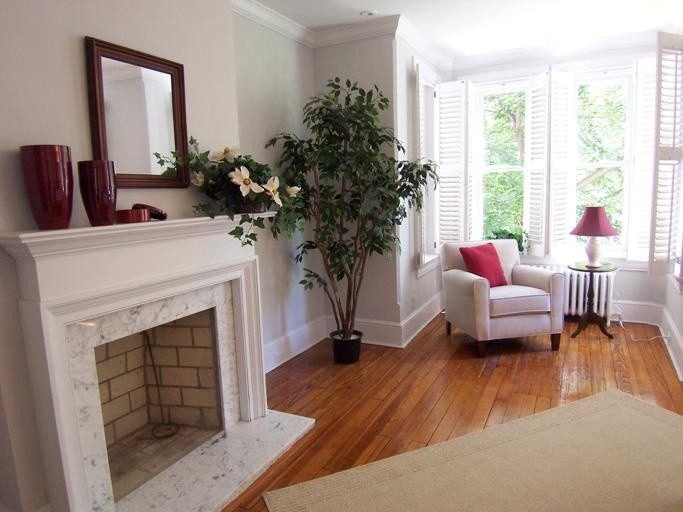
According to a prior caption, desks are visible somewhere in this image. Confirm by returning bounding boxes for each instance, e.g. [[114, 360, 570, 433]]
[[567, 261, 616, 340]]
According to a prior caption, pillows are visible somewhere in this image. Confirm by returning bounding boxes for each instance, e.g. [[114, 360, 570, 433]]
[[459, 242, 507, 288]]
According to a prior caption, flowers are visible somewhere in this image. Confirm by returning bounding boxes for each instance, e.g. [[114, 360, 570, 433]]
[[184, 137, 311, 246]]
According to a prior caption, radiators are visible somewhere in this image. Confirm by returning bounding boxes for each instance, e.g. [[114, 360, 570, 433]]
[[530, 264, 616, 328]]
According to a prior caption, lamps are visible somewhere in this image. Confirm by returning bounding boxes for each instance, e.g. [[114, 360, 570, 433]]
[[569, 206, 618, 267]]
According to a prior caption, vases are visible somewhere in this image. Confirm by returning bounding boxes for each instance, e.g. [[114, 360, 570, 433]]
[[20, 144, 73, 229], [78, 160, 117, 226], [237, 203, 261, 214]]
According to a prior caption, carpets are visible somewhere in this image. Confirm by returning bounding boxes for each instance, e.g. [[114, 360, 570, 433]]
[[261, 389, 683, 512]]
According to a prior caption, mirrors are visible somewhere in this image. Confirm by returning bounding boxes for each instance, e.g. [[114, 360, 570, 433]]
[[84, 35, 189, 188]]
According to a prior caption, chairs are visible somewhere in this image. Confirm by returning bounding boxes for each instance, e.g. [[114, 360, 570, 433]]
[[440, 239, 564, 357]]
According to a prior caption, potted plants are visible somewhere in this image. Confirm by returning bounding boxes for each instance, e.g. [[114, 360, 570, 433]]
[[266, 76, 439, 365]]
